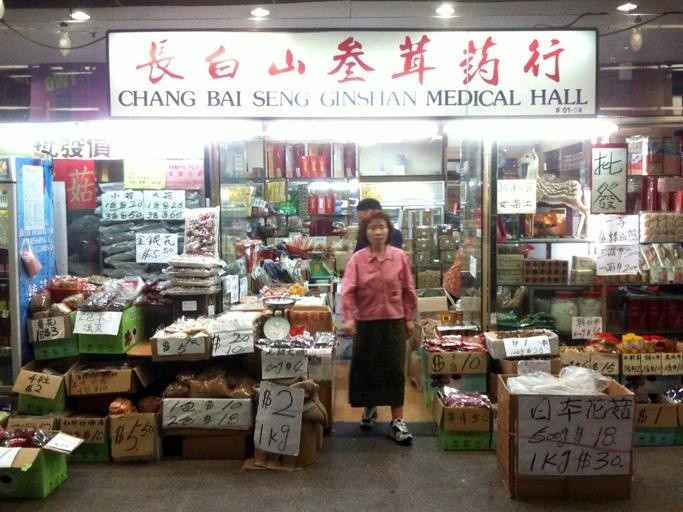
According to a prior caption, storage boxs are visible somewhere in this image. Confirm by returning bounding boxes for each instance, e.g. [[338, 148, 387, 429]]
[[159, 394, 256, 438], [209, 309, 266, 351], [2, 306, 15, 411], [260, 350, 334, 438], [181, 434, 247, 459], [664, 392, 682, 446], [151, 317, 212, 361], [109, 412, 169, 462], [60, 413, 111, 463], [490, 410, 496, 451], [11, 360, 66, 416], [409, 287, 682, 408], [1, 417, 87, 498], [71, 306, 148, 354], [496, 372, 636, 499], [432, 391, 492, 452], [63, 356, 154, 413], [26, 310, 81, 360], [632, 391, 678, 447]]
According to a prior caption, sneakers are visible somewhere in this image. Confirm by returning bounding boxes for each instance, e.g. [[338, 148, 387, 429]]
[[360, 407, 377, 428], [384, 418, 412, 444]]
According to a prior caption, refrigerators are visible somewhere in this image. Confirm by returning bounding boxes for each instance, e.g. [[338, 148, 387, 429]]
[[0, 155, 57, 408]]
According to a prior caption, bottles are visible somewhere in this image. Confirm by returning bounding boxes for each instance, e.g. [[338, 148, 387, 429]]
[[0, 191, 8, 217], [0, 282, 6, 310], [549, 291, 602, 334], [0, 160, 7, 176]]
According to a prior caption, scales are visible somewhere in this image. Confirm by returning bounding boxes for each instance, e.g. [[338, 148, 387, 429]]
[[308, 252, 334, 280], [262, 297, 296, 342]]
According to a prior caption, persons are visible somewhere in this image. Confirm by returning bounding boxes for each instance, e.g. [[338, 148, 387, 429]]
[[340, 199, 417, 444]]
